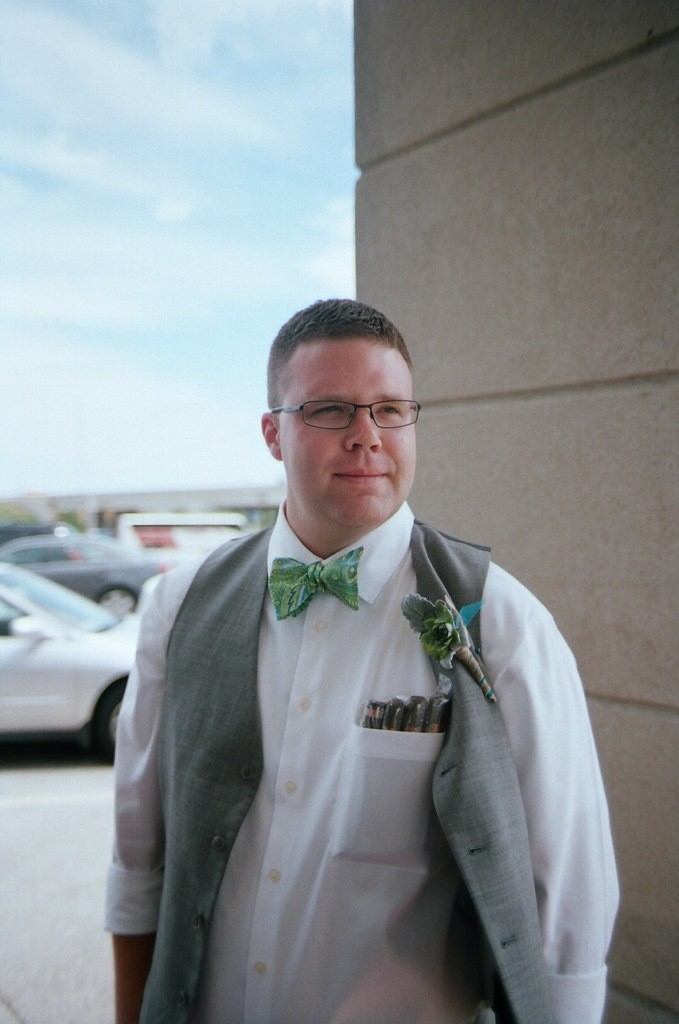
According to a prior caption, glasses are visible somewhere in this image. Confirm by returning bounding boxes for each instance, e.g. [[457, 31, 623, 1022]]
[[271, 400, 421, 429]]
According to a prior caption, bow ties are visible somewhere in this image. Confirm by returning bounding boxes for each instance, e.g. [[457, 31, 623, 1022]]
[[267, 544, 365, 621]]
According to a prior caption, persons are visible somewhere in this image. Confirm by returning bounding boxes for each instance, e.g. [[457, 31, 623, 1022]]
[[103, 300, 620, 1023]]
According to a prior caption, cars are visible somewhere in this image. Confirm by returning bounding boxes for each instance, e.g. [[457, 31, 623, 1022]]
[[1, 536, 172, 621], [0, 561, 143, 764]]
